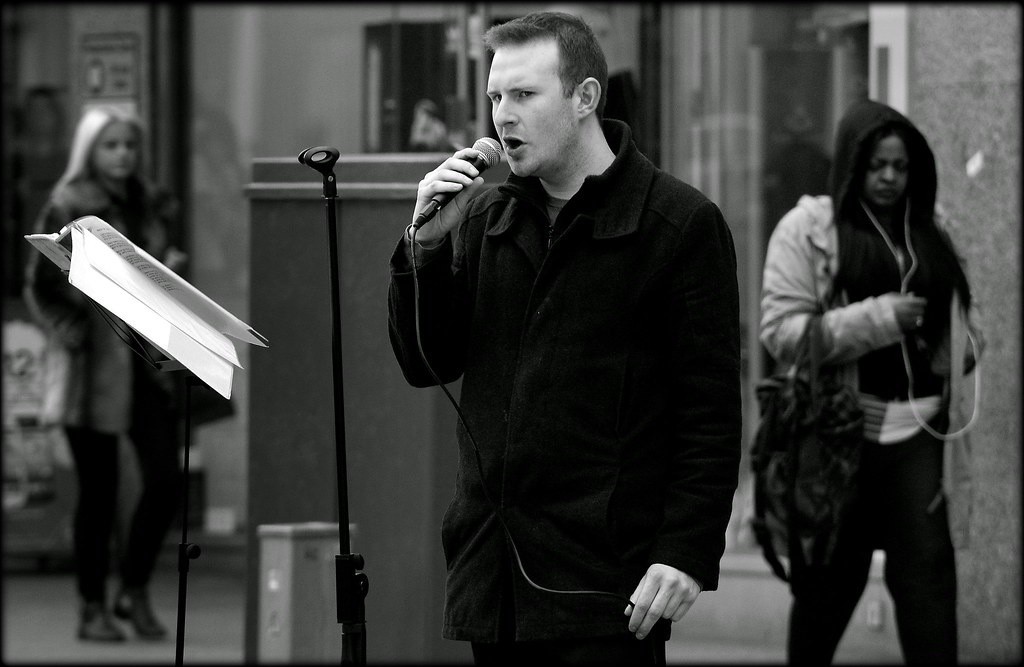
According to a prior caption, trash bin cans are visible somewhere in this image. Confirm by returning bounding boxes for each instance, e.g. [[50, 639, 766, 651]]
[[256, 523, 357, 667]]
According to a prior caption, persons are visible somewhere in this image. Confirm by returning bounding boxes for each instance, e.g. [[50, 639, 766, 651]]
[[388, 12, 744, 667], [23, 100, 189, 642], [759, 101, 984, 667]]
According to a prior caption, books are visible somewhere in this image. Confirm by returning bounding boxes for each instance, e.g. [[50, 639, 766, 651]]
[[24, 217, 268, 401]]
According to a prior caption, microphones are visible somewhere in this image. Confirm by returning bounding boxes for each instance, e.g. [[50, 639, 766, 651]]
[[414, 137, 500, 227]]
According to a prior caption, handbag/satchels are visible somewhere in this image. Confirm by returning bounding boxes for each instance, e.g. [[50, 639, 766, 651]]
[[750, 315, 866, 584]]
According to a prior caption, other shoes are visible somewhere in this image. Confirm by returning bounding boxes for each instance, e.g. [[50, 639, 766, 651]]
[[114, 591, 168, 638], [76, 608, 126, 642]]
